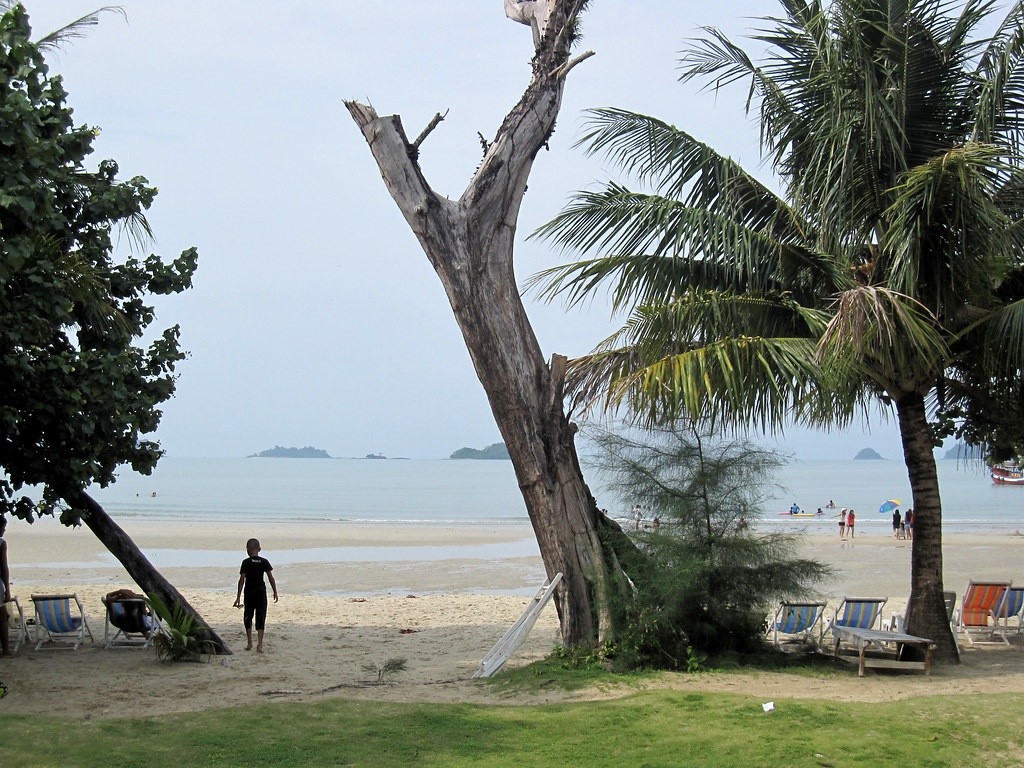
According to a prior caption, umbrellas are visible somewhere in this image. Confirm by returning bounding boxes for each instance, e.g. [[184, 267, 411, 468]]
[[878, 499, 902, 513]]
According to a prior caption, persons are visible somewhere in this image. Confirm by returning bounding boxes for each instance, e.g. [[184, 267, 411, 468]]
[[790, 503, 800, 514], [846, 510, 855, 537], [817, 508, 823, 513], [602, 509, 607, 515], [634, 505, 644, 530], [107, 590, 161, 628], [829, 500, 834, 506], [233, 539, 278, 652], [833, 508, 847, 537], [737, 518, 749, 529], [893, 509, 901, 539], [0, 515, 12, 655], [904, 509, 914, 540]]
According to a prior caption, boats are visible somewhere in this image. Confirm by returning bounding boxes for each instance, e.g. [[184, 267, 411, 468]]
[[988, 460, 1024, 487]]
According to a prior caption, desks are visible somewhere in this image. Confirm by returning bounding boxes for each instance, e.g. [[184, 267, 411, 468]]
[[832, 624, 936, 678]]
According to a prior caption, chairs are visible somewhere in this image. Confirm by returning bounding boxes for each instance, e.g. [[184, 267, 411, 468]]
[[818, 595, 890, 658], [884, 580, 1024, 649], [4, 596, 33, 653], [762, 601, 827, 655], [29, 592, 95, 652], [101, 594, 167, 654]]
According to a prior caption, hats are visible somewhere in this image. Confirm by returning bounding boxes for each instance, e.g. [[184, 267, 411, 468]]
[[841, 507, 847, 512]]
